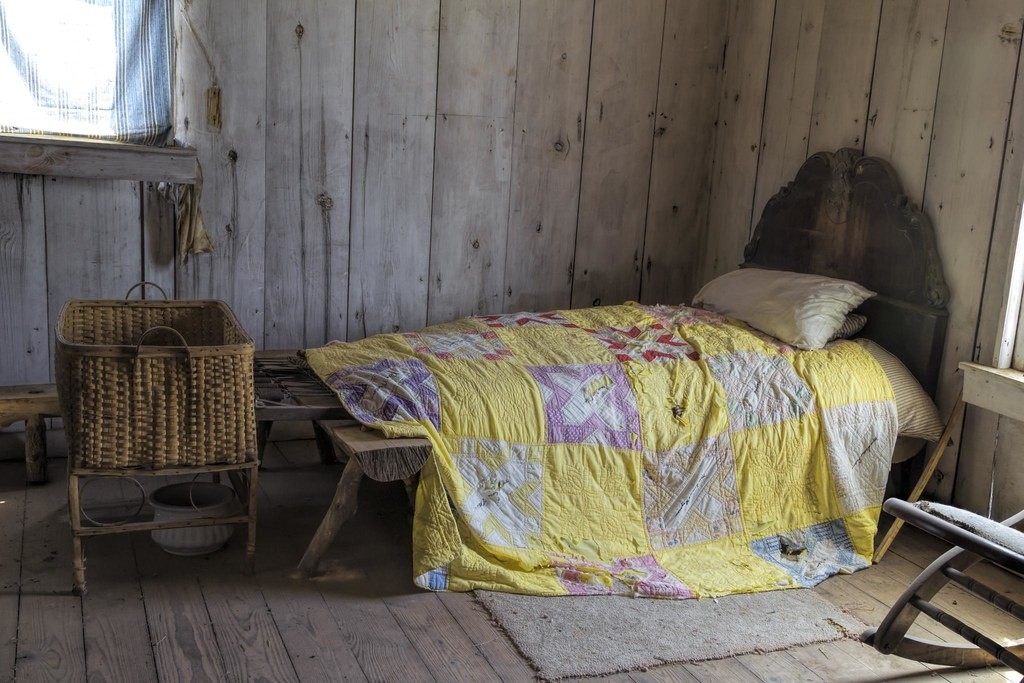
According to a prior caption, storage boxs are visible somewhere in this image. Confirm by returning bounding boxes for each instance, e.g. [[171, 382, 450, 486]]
[[54, 284, 263, 468]]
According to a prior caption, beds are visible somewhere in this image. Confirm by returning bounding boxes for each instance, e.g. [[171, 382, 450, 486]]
[[280, 299, 954, 584]]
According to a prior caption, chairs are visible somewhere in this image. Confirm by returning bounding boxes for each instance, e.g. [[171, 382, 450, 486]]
[[860, 483, 1024, 683]]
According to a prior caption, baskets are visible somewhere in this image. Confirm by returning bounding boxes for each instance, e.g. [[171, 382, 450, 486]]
[[54, 280, 258, 470]]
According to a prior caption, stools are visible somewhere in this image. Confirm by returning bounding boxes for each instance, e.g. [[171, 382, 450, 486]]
[[0, 381, 65, 482], [62, 442, 262, 595]]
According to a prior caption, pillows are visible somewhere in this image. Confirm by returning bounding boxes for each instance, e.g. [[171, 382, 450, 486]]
[[815, 312, 872, 345], [689, 267, 877, 348]]
